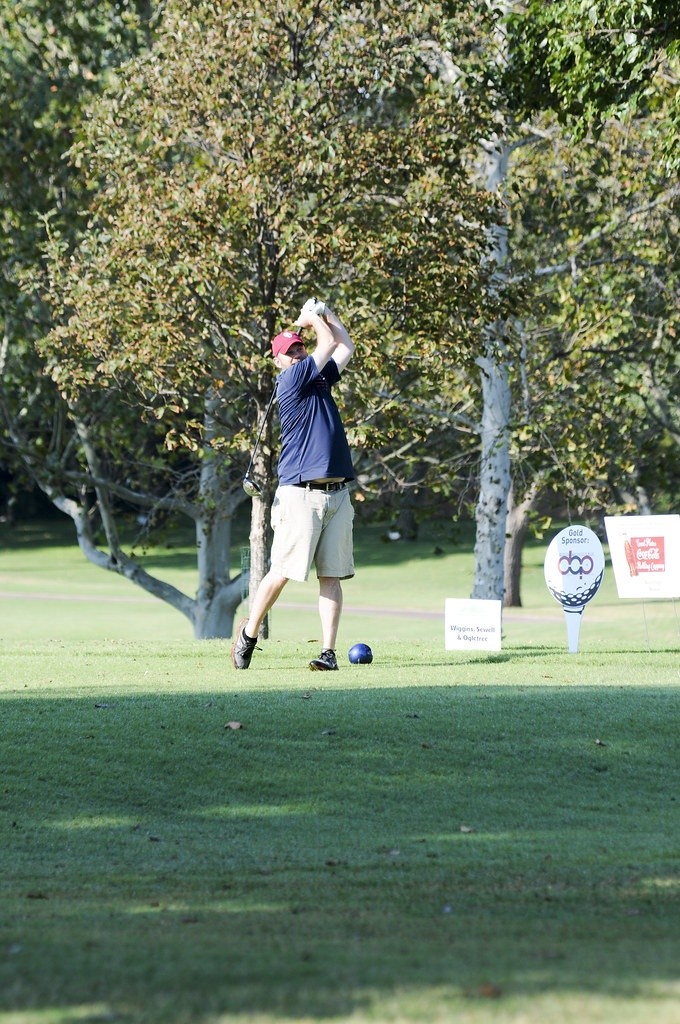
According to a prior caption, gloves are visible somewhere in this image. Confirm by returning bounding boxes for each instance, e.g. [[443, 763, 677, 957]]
[[303, 298, 326, 316]]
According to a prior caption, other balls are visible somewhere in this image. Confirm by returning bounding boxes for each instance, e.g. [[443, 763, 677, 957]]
[[347, 643, 373, 664]]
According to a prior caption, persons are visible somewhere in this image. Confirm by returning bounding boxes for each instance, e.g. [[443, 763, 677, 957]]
[[232, 308, 356, 670]]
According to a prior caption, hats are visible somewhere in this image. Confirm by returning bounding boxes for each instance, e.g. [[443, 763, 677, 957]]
[[272, 331, 305, 357]]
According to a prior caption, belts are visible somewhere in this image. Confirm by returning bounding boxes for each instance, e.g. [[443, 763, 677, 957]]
[[293, 480, 346, 491]]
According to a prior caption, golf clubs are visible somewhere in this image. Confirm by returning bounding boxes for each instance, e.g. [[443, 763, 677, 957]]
[[243, 295, 318, 497]]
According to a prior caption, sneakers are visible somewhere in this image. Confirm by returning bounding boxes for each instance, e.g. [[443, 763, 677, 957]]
[[308, 648, 340, 671], [232, 617, 257, 669]]
